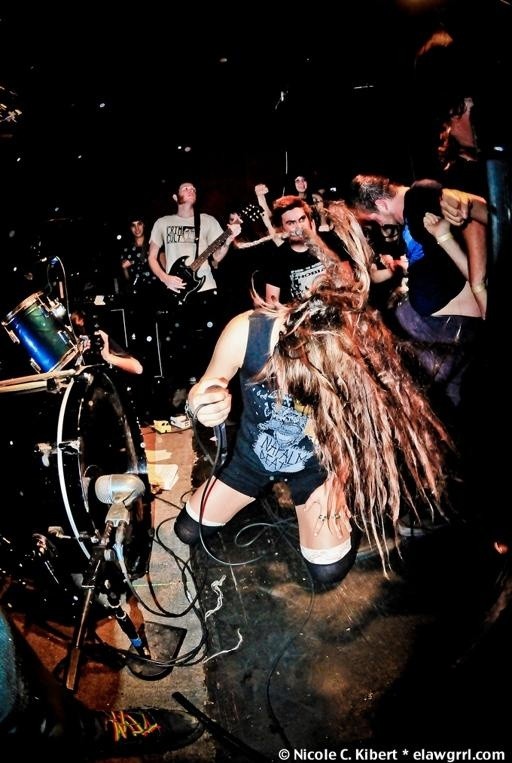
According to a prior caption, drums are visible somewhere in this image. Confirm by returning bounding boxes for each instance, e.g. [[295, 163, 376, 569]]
[[1, 363, 153, 627], [2, 290, 82, 374]]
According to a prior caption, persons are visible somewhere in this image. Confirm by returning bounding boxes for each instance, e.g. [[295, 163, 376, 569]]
[[1, 91, 512, 761]]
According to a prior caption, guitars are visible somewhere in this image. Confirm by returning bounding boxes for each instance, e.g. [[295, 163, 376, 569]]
[[143, 204, 265, 314]]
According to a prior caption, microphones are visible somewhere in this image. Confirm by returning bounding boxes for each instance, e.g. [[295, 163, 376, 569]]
[[93, 472, 147, 506]]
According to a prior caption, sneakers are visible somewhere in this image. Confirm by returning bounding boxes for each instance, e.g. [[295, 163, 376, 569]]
[[91, 705, 206, 759]]
